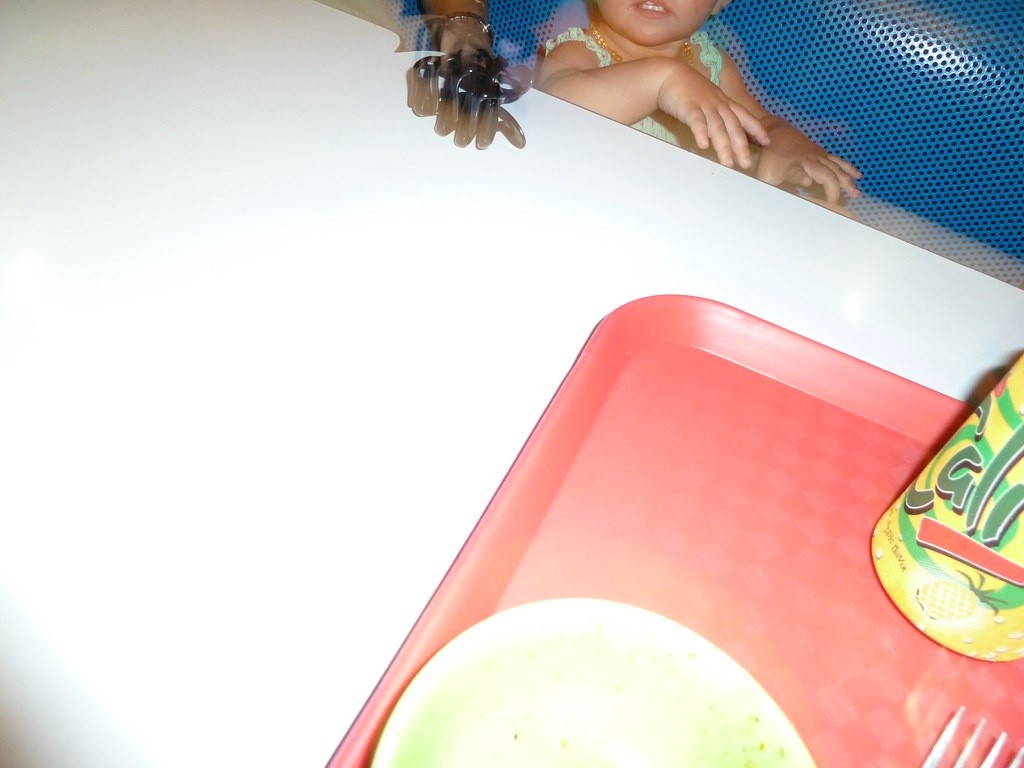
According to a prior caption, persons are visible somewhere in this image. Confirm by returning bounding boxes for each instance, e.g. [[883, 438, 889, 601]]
[[422, 0, 864, 219]]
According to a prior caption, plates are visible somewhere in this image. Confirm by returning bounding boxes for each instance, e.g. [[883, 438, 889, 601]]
[[370, 598, 816, 768]]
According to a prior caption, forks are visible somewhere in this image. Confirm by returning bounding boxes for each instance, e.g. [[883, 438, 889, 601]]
[[921, 706, 1024, 768]]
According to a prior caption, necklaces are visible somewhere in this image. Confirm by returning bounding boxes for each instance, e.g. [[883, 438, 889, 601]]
[[590, 22, 694, 67]]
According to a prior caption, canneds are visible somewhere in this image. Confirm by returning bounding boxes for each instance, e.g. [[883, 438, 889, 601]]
[[870, 349, 1024, 665]]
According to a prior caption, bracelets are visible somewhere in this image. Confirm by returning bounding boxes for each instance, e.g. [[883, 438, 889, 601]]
[[436, 12, 494, 47]]
